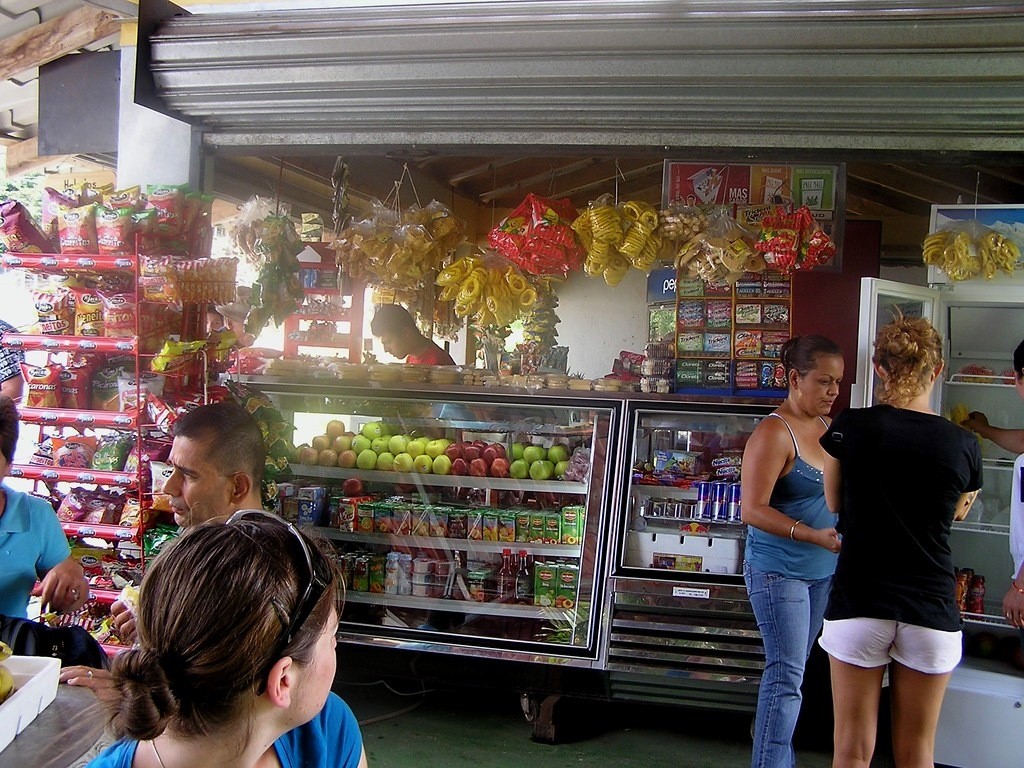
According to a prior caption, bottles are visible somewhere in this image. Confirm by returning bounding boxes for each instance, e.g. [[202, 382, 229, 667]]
[[336, 551, 383, 593], [497, 549, 545, 604], [953, 566, 985, 621], [385, 552, 411, 595], [467, 561, 486, 571]]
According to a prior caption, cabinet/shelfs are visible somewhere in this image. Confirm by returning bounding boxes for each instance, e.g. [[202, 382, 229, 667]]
[[280, 264, 368, 362], [616, 399, 791, 585], [732, 269, 794, 398], [672, 269, 732, 397], [279, 372, 596, 661], [0, 223, 223, 658]]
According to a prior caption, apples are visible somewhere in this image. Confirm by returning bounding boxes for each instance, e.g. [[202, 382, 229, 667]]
[[294, 419, 572, 481], [342, 479, 362, 496]]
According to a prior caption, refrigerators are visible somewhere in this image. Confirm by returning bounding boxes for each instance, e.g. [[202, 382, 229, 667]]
[[851, 204, 1024, 768]]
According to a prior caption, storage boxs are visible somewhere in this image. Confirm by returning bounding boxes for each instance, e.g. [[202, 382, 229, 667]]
[[253, 397, 753, 621]]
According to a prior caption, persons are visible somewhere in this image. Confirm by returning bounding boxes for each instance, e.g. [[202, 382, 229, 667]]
[[82, 510, 367, 768], [741, 335, 845, 768], [0, 320, 25, 406], [0, 394, 90, 619], [206, 303, 255, 349], [58, 402, 266, 701], [817, 317, 984, 768], [371, 304, 459, 438], [958, 338, 1024, 629]]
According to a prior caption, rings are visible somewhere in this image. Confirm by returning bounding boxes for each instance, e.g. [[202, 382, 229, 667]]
[[71, 589, 78, 595], [88, 670, 94, 677], [1006, 613, 1012, 619]]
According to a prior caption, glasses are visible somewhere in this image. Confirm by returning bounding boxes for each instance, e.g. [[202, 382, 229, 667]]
[[225, 507, 331, 695]]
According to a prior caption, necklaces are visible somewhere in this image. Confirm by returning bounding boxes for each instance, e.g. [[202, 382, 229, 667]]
[[149, 740, 166, 768]]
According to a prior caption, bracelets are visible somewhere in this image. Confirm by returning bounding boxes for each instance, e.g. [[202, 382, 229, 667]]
[[1012, 581, 1024, 594], [790, 520, 803, 543]]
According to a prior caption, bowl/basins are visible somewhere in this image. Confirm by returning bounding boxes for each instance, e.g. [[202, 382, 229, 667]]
[[413, 557, 449, 597]]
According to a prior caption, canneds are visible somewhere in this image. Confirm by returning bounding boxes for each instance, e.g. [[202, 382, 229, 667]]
[[696, 482, 743, 524], [336, 551, 388, 593]]
